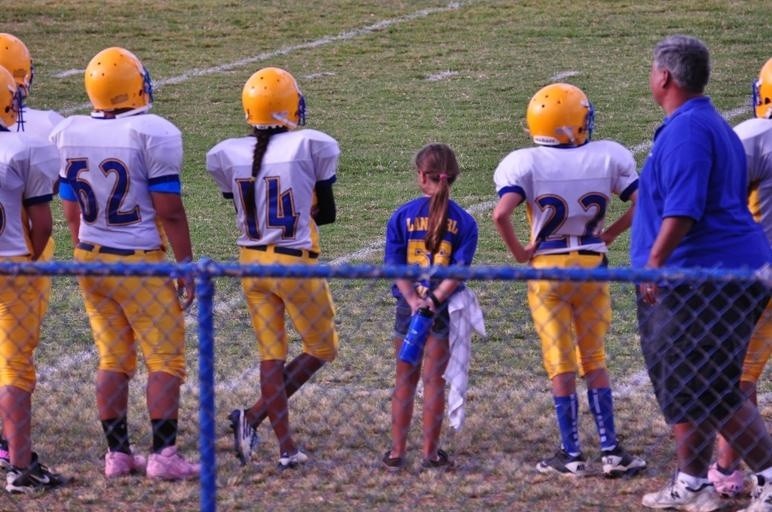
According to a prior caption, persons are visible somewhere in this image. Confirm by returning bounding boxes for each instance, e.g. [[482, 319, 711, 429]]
[[49, 46, 201, 481], [0, 66, 64, 495], [706, 56, 771, 498], [628, 36, 772, 512], [382, 144, 478, 469], [0, 32, 67, 469], [206, 67, 341, 469], [492, 82, 647, 478]]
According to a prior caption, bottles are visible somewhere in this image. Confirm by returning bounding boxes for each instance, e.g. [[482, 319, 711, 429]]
[[397, 305, 435, 368]]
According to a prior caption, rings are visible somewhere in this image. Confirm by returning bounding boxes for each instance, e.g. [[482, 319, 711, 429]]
[[646, 288, 652, 292]]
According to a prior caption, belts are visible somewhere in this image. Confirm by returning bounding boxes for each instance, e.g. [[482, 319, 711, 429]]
[[246, 245, 319, 259], [78, 242, 148, 255], [545, 248, 602, 256]]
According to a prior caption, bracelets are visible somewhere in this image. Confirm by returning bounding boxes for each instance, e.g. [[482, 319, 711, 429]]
[[436, 285, 450, 298], [402, 285, 414, 298], [427, 291, 441, 309]]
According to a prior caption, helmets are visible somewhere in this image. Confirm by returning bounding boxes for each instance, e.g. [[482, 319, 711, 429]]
[[755, 57, 772, 117], [0, 32, 32, 87], [85, 47, 149, 110], [526, 83, 589, 145], [0, 64, 18, 125], [242, 66, 300, 128]]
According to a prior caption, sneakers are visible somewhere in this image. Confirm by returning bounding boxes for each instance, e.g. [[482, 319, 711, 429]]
[[738, 466, 772, 512], [708, 461, 744, 498], [104, 451, 146, 476], [601, 445, 647, 477], [146, 445, 201, 480], [278, 450, 307, 466], [423, 449, 449, 469], [536, 450, 586, 477], [5, 451, 61, 494], [382, 452, 402, 471], [0, 439, 9, 468], [642, 481, 721, 512], [232, 407, 258, 466]]
